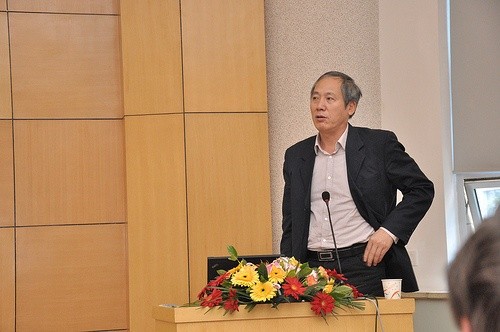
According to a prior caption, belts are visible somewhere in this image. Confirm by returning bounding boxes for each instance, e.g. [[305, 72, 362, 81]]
[[307, 243, 368, 261]]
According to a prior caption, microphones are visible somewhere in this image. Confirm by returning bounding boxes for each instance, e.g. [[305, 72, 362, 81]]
[[322, 192, 342, 275]]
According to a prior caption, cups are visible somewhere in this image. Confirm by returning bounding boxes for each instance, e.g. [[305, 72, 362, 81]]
[[381, 279, 402, 300]]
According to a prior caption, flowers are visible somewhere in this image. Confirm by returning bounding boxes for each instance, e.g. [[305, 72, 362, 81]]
[[175, 245, 367, 327]]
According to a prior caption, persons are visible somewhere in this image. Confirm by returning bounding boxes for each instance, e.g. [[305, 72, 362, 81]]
[[446, 208, 500, 332], [279, 70, 435, 299]]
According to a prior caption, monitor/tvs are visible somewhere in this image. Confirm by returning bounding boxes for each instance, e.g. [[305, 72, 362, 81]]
[[207, 255, 284, 284]]
